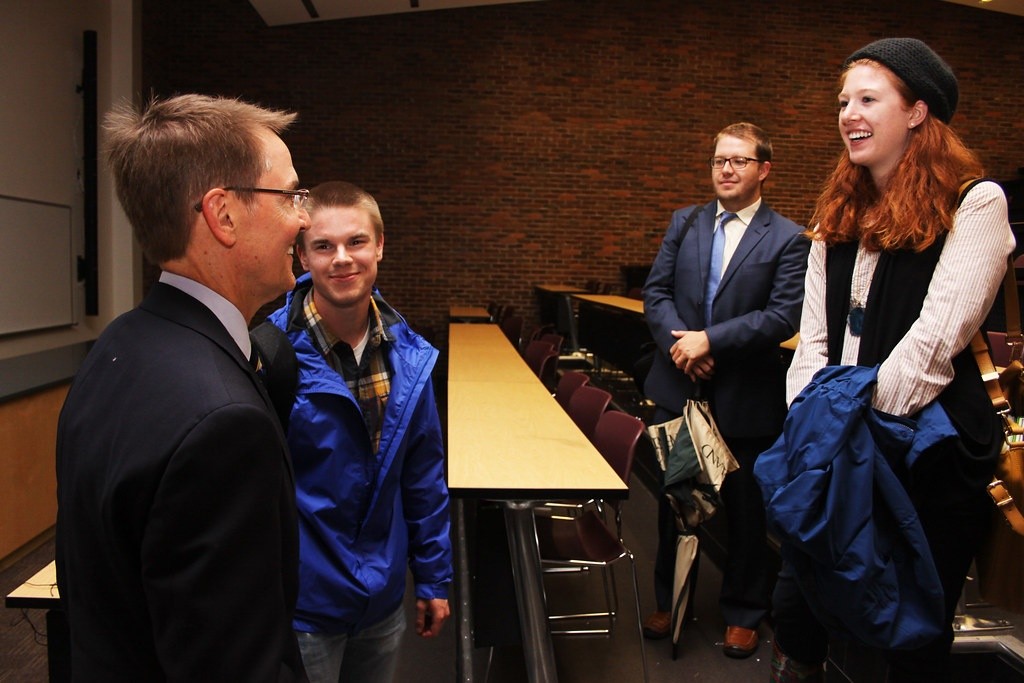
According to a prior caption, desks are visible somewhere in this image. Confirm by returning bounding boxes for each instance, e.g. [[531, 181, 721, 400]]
[[444, 280, 805, 683]]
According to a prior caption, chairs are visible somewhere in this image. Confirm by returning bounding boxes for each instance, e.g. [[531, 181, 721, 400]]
[[486, 263, 705, 683]]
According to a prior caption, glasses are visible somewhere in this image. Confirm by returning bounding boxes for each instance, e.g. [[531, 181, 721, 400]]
[[194, 189, 309, 212], [703, 211, 738, 328], [709, 156, 764, 169]]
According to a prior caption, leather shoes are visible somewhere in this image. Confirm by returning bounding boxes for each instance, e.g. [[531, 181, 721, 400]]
[[723, 625, 760, 658], [643, 605, 693, 640]]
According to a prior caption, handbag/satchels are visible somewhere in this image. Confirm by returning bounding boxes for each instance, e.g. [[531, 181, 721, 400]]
[[975, 359, 1024, 623]]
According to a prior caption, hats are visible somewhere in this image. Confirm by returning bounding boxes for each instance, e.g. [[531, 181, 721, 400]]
[[843, 38, 959, 126]]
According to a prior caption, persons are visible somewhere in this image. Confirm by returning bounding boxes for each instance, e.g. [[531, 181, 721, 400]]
[[55, 95, 312, 683], [643, 122, 809, 657], [248, 180, 453, 683], [786, 37, 1018, 683]]
